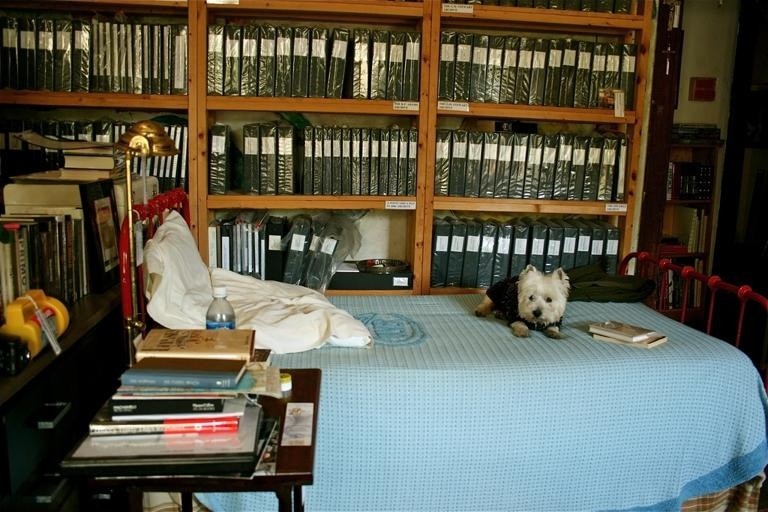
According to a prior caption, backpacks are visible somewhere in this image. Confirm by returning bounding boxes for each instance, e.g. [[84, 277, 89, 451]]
[[566, 260, 657, 304]]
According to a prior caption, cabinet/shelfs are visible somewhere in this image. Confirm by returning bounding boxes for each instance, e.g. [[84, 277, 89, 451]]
[[639, 122, 723, 323], [1, 149, 167, 512]]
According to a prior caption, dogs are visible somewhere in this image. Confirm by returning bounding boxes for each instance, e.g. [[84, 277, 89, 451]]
[[474, 262, 571, 339]]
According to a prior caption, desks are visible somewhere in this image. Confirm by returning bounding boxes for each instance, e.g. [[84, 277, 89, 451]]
[[61, 366, 321, 511]]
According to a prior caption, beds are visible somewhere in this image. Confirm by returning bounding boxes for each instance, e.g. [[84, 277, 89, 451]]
[[114, 186, 767, 512]]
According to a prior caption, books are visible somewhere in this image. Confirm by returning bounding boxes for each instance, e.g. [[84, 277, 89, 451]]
[[0, 144, 126, 321], [37, 398, 72, 430], [45, 327, 285, 482], [591, 333, 666, 350], [32, 475, 68, 505], [587, 320, 655, 342]]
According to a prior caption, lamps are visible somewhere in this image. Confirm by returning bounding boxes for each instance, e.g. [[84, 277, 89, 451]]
[[110, 120, 184, 368]]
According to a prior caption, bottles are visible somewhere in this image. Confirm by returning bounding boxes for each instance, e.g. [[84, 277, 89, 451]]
[[204, 284, 237, 330]]
